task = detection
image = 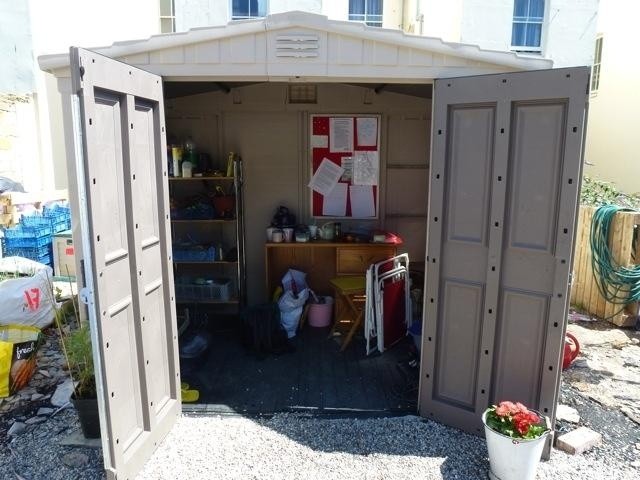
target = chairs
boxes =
[366,253,412,355]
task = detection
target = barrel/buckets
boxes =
[308,296,332,327]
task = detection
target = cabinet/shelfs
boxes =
[265,230,397,332]
[169,160,246,304]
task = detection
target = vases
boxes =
[482,406,551,480]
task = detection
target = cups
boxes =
[272,230,285,243]
[283,228,293,242]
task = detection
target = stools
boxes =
[329,277,366,353]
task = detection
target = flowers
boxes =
[487,400,548,438]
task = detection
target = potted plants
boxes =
[61,320,101,439]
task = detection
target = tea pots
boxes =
[318,222,333,239]
